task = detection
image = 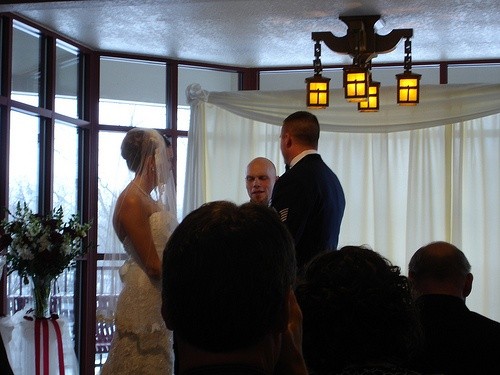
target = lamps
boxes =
[304,16,422,113]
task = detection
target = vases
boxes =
[33,287,51,318]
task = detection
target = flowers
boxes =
[184,83,210,107]
[0,202,94,286]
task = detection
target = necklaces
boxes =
[130,178,158,206]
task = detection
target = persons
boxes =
[409,242,499,375]
[245,157,281,208]
[295,246,427,374]
[269,111,346,277]
[99,126,179,375]
[160,202,309,375]
